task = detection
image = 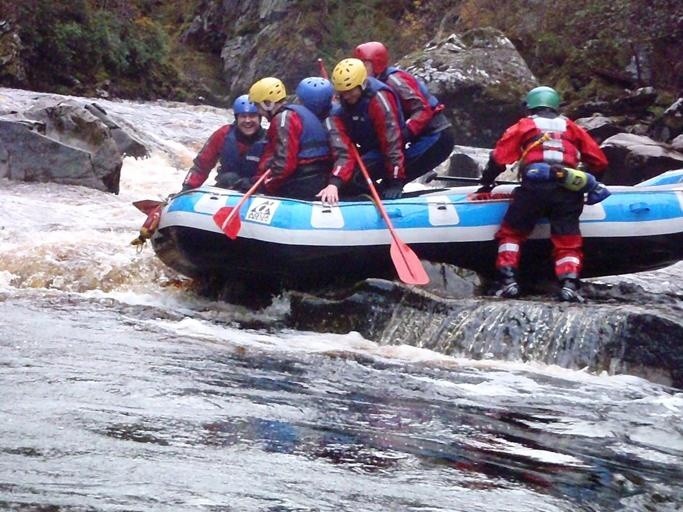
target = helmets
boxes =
[248,77,287,103]
[295,76,334,117]
[355,42,389,74]
[526,85,560,111]
[331,57,369,91]
[232,94,258,115]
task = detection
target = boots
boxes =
[560,278,585,303]
[481,266,520,298]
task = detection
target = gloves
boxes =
[381,179,403,199]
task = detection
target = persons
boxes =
[164,94,271,199]
[477,86,608,304]
[355,42,456,200]
[332,58,406,201]
[248,77,334,201]
[296,77,357,204]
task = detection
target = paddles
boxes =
[132,200,163,217]
[356,154,430,285]
[212,168,272,240]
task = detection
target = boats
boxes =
[147,166,683,297]
